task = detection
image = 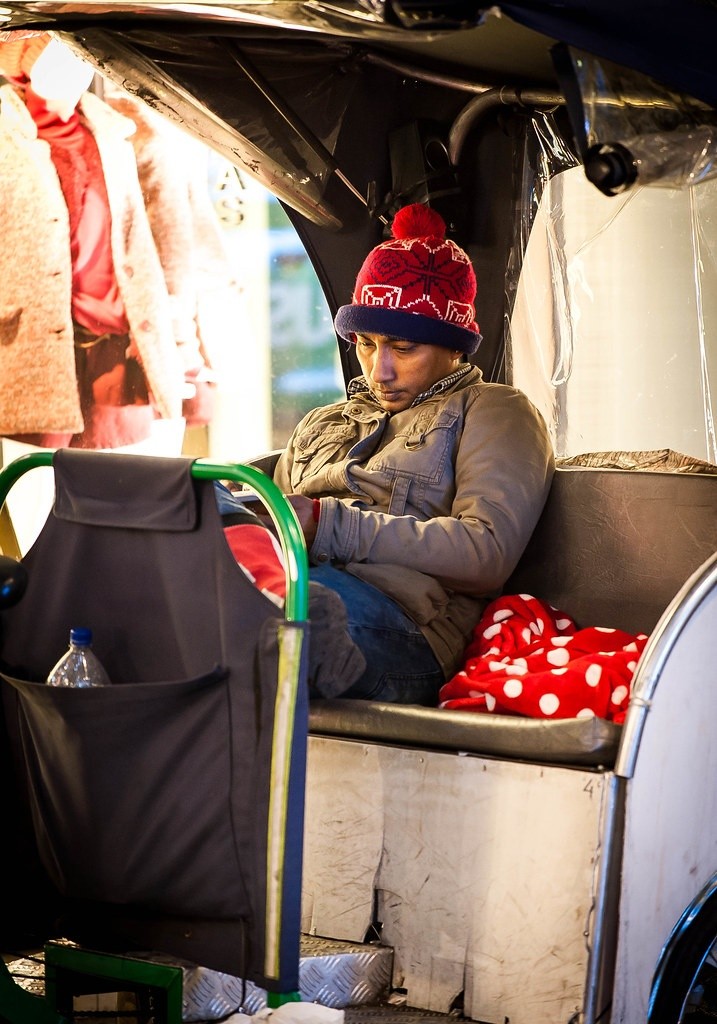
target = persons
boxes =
[223,201,555,705]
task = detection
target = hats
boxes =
[334,204,483,355]
[0,27,53,79]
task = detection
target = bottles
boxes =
[45,627,109,686]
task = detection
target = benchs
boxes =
[305,470,716,763]
